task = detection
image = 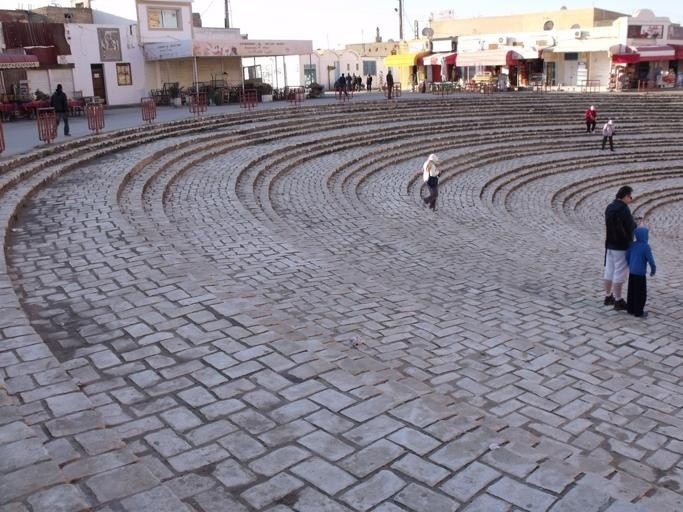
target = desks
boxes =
[0,99,82,121]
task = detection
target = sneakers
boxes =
[603,295,613,306]
[614,299,627,310]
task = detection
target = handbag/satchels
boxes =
[427,176,439,188]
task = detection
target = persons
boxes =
[386,70,392,99]
[33,90,49,118]
[50,84,72,136]
[337,73,372,97]
[584,105,597,135]
[600,119,616,151]
[625,226,657,318]
[422,154,441,210]
[603,186,641,310]
